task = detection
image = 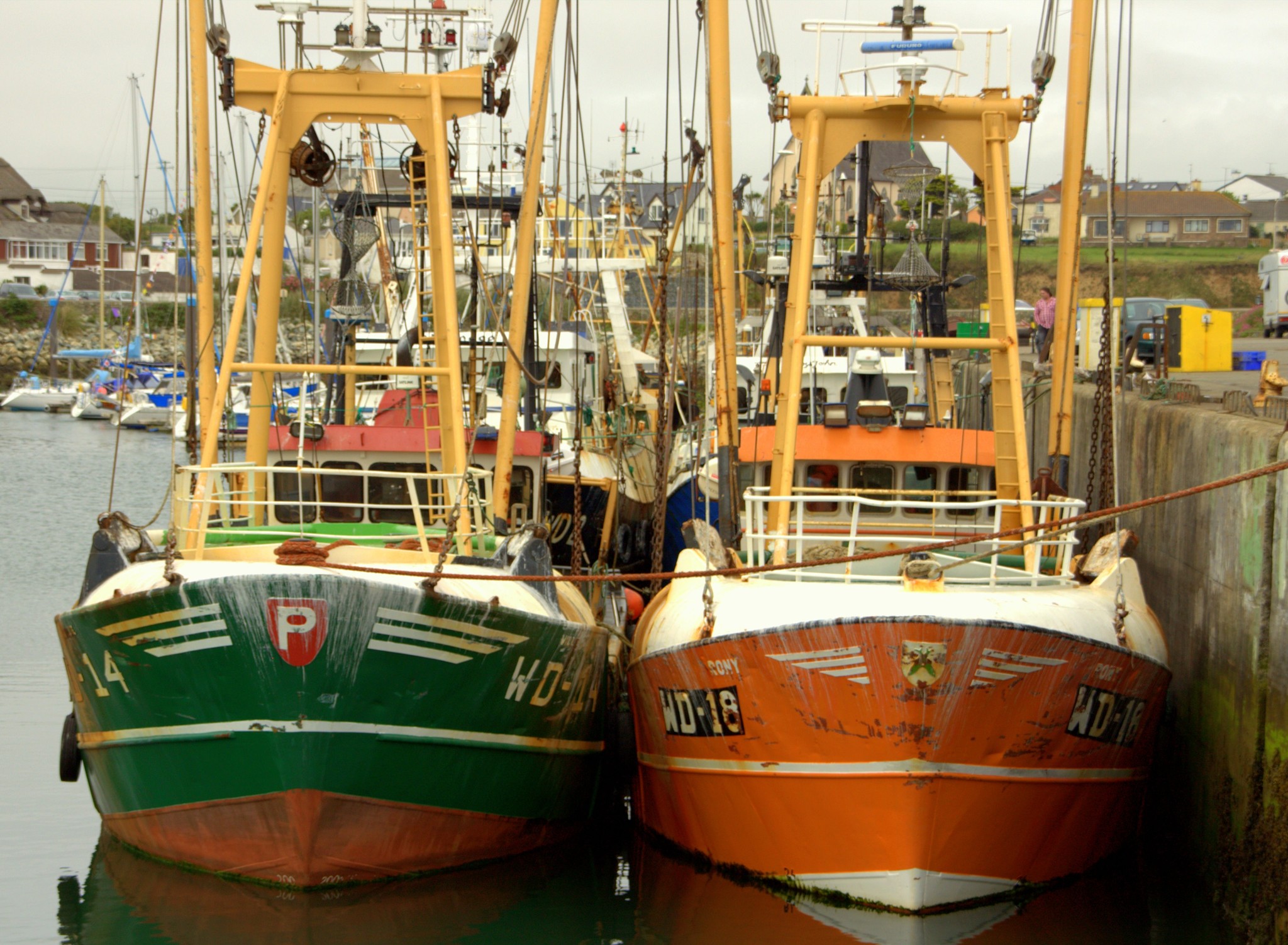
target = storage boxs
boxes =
[1233,351,1266,371]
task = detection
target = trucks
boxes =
[1257,249,1288,339]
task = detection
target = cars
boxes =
[1168,298,1211,310]
[1019,229,1037,246]
[1014,298,1039,339]
[1118,298,1175,367]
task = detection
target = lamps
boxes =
[821,402,849,426]
[742,270,764,284]
[850,153,856,170]
[474,424,497,438]
[334,25,350,45]
[901,403,930,428]
[737,364,755,386]
[949,274,978,288]
[289,421,324,441]
[366,26,382,45]
[856,400,891,424]
[826,289,843,296]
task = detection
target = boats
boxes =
[622,0,1174,915]
[49,0,650,890]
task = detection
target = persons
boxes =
[1034,287,1057,362]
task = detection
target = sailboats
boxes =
[0,71,928,584]
